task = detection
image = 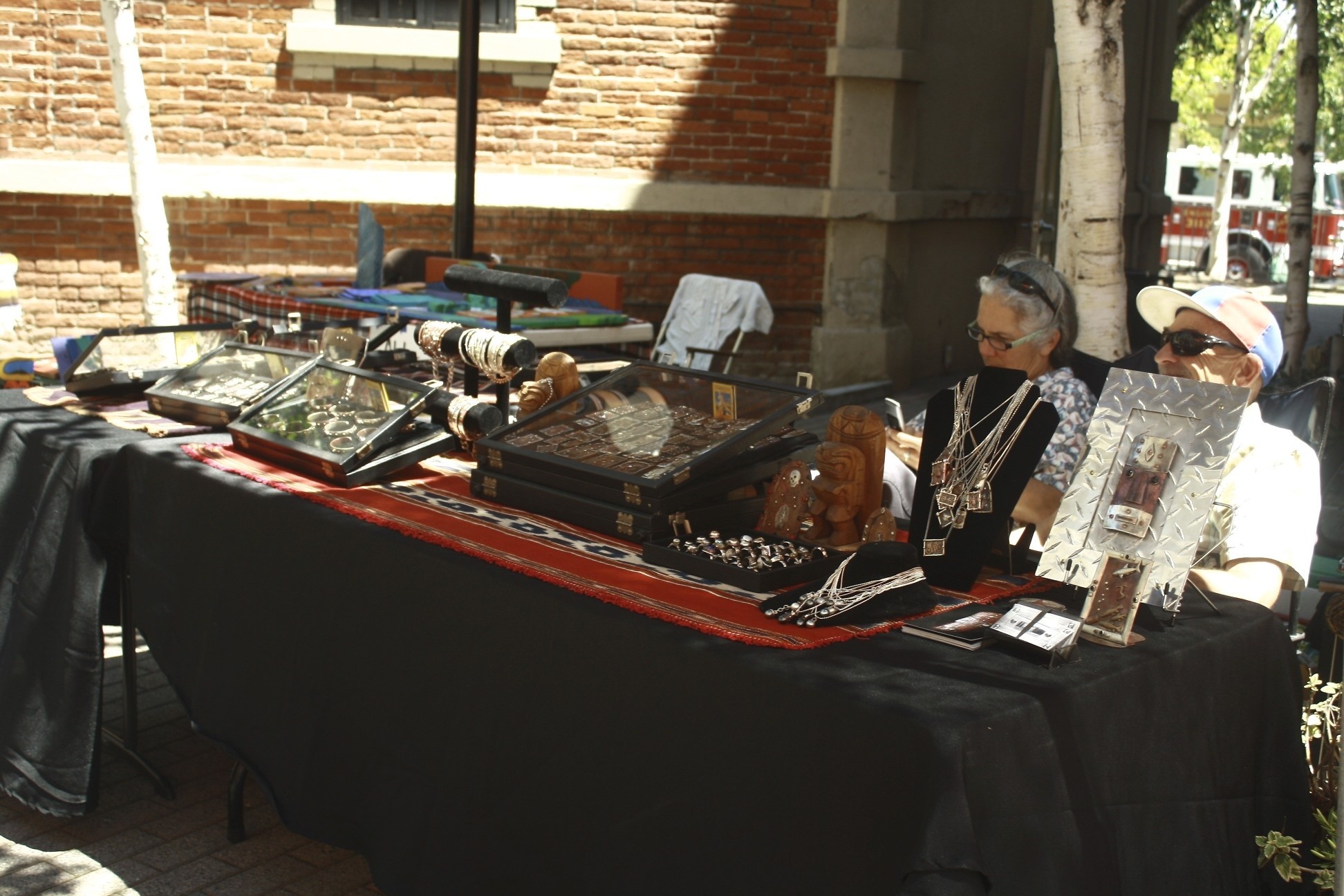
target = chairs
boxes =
[1062,343,1344,806]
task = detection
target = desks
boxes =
[96,434,1308,896]
[187,282,655,356]
[1,381,220,822]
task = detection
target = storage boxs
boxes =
[63,320,859,594]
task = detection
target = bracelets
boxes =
[418,318,528,392]
[253,396,387,453]
[447,395,487,450]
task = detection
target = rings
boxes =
[668,531,828,572]
[903,451,909,463]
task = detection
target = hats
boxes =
[1136,284,1285,388]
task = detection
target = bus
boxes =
[1156,144,1344,289]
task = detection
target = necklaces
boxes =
[765,552,926,629]
[922,375,1042,556]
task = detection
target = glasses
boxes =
[966,321,1053,352]
[983,263,1058,318]
[1159,328,1249,356]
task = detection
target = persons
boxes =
[1136,283,1322,608]
[883,249,1097,546]
